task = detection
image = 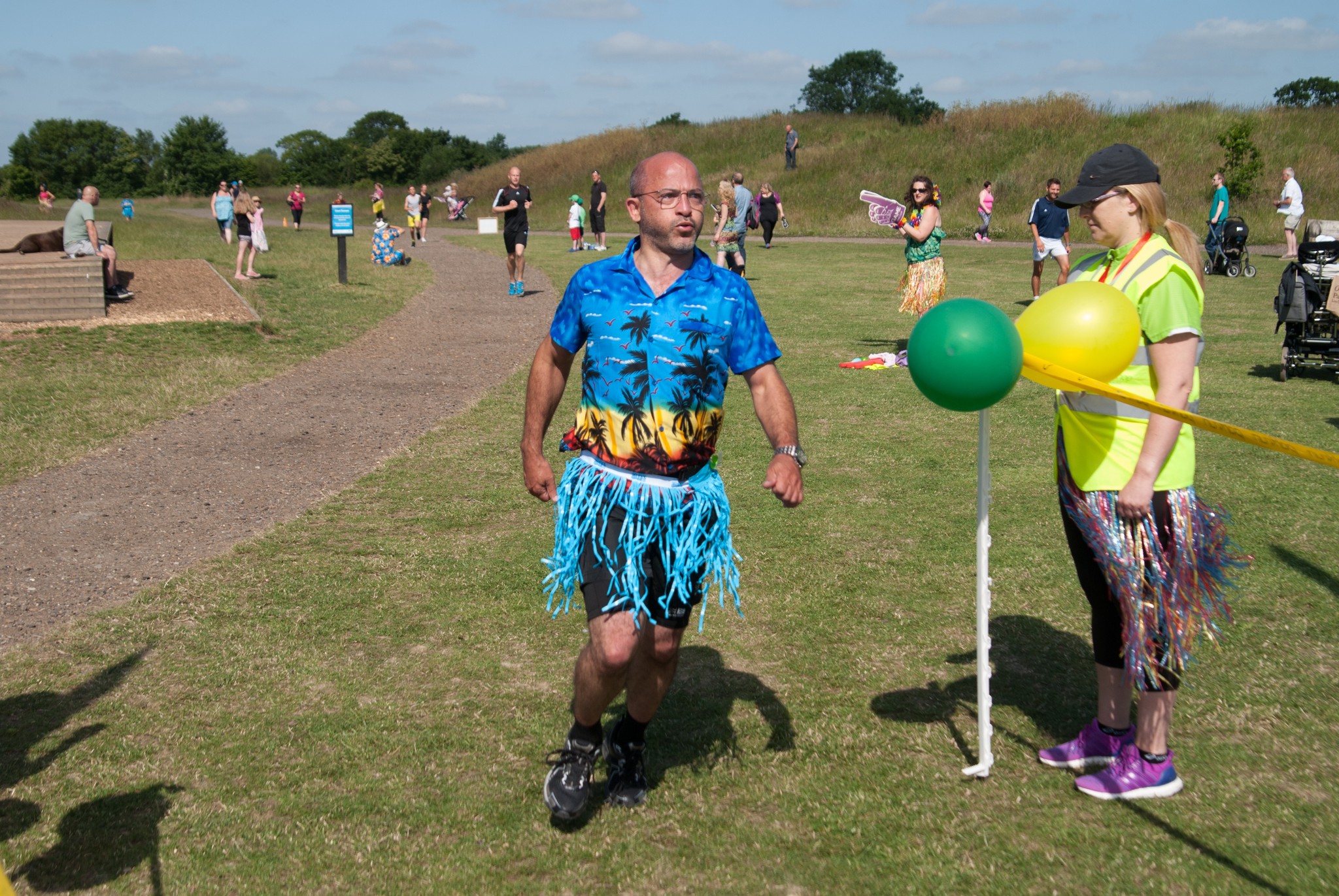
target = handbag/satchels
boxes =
[250,217,268,252]
[1273,274,1313,322]
[286,193,294,206]
[372,199,385,214]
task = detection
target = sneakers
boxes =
[600,723,647,807]
[515,281,524,296]
[975,232,982,241]
[1279,254,1294,260]
[105,281,134,298]
[509,282,516,295]
[982,237,991,242]
[1073,743,1183,799]
[544,723,605,818]
[1293,254,1298,259]
[1038,717,1138,767]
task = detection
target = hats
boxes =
[230,180,262,203]
[374,220,388,232]
[1054,142,1162,209]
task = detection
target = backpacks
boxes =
[746,191,761,229]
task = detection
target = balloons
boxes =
[907,297,1023,412]
[1015,280,1141,393]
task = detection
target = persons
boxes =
[975,181,995,241]
[1273,167,1305,260]
[38,183,136,298]
[1205,172,1229,272]
[784,124,799,170]
[1027,178,1072,300]
[589,170,607,251]
[491,166,532,297]
[211,179,464,280]
[521,152,806,820]
[1036,143,1247,801]
[711,172,784,279]
[859,175,946,321]
[567,194,586,252]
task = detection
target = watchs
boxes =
[1066,242,1071,245]
[774,445,807,468]
[95,248,100,253]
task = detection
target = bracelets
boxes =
[1278,199,1280,204]
[899,217,908,228]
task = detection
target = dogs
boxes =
[0,226,65,256]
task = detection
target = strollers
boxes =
[1272,240,1339,381]
[1203,215,1257,278]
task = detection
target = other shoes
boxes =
[373,220,378,224]
[246,270,261,277]
[234,273,248,279]
[766,243,771,248]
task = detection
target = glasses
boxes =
[1213,178,1221,181]
[220,184,227,186]
[634,188,710,206]
[1082,189,1129,208]
[911,188,930,193]
